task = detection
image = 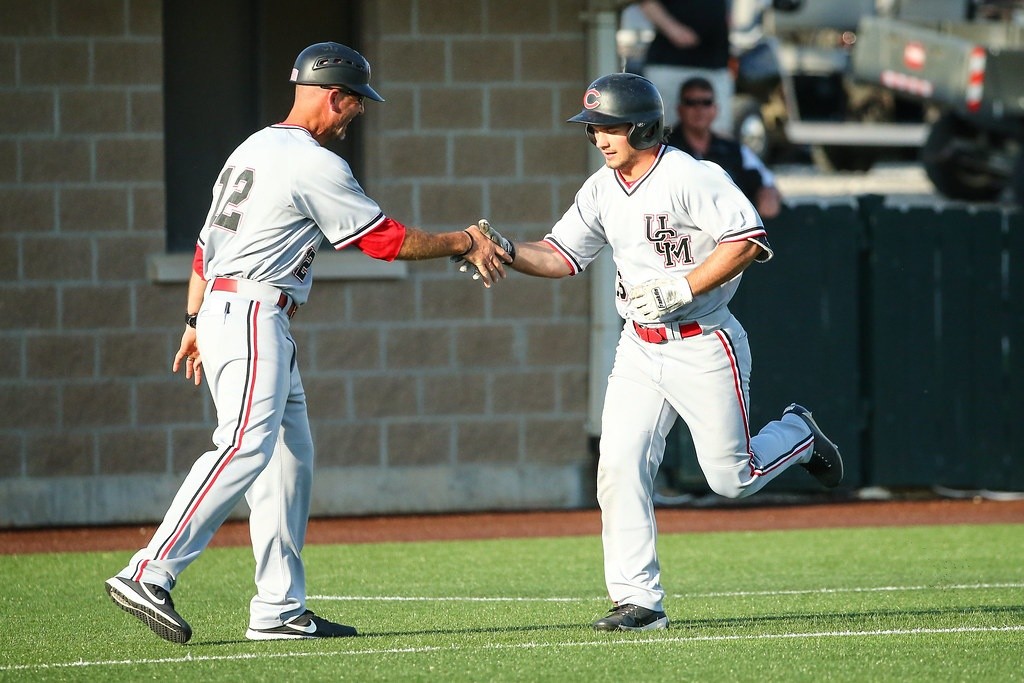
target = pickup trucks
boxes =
[848,11,1024,201]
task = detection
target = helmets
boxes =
[567,72,664,149]
[289,41,384,106]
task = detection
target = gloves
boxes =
[448,218,514,281]
[629,277,696,320]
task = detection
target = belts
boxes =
[211,278,296,318]
[633,321,700,344]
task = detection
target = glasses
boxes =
[683,98,713,108]
[318,81,366,104]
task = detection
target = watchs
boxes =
[185,311,198,329]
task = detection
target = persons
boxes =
[105,41,513,644]
[640,1,735,137]
[664,76,781,215]
[449,74,843,630]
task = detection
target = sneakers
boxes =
[783,404,843,487]
[244,613,356,642]
[595,601,669,632]
[105,576,192,644]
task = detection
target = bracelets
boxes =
[457,230,474,256]
